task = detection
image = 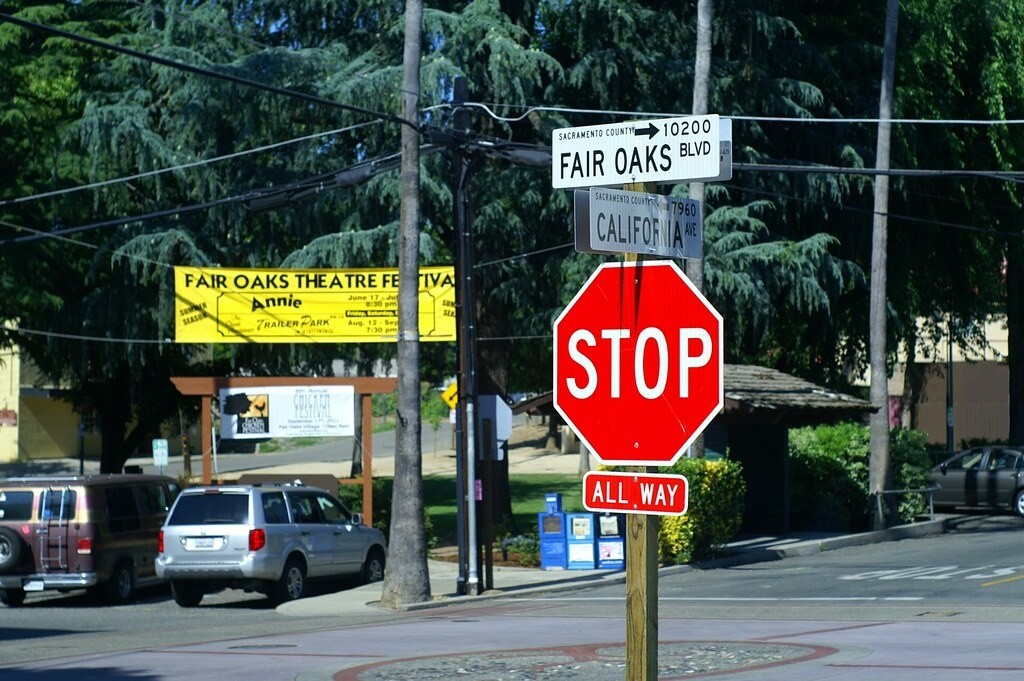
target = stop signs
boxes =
[552,259,725,467]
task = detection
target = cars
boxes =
[923,444,1024,519]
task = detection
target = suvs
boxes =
[157,480,388,609]
[0,473,184,607]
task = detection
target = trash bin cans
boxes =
[538,512,624,569]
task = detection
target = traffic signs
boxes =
[572,186,705,259]
[551,114,720,189]
